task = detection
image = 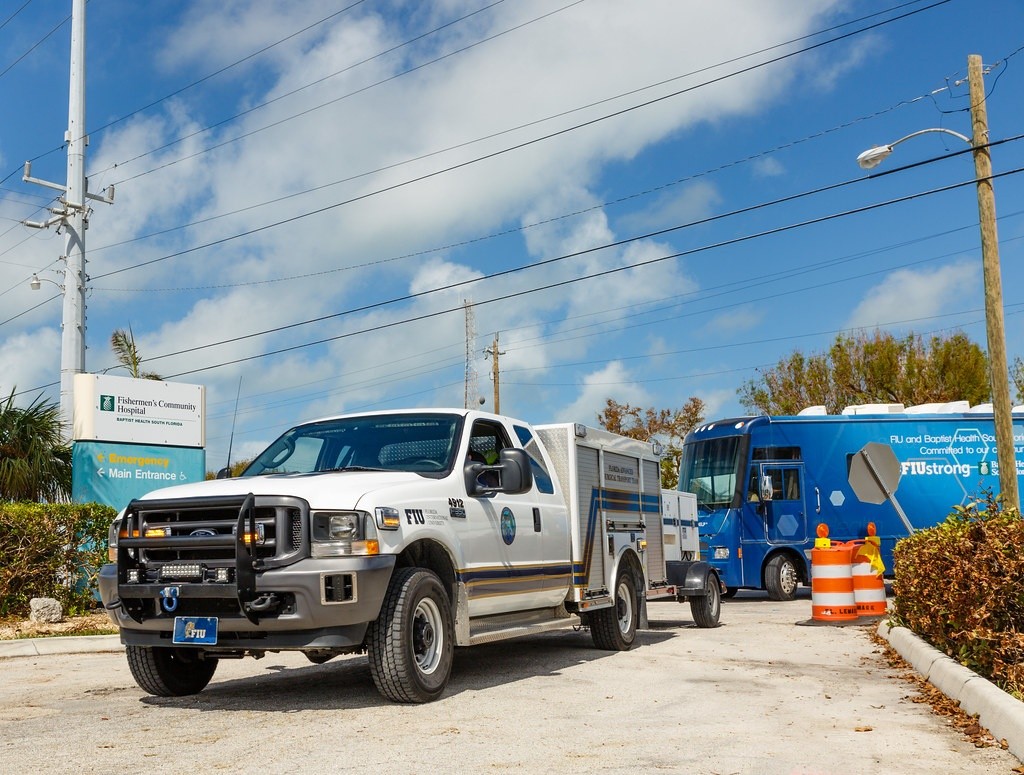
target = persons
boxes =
[437,443,488,487]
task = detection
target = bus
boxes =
[677,392,1024,601]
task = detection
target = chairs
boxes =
[471,451,499,498]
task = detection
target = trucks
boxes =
[94,409,725,704]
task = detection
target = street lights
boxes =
[856,128,1023,515]
